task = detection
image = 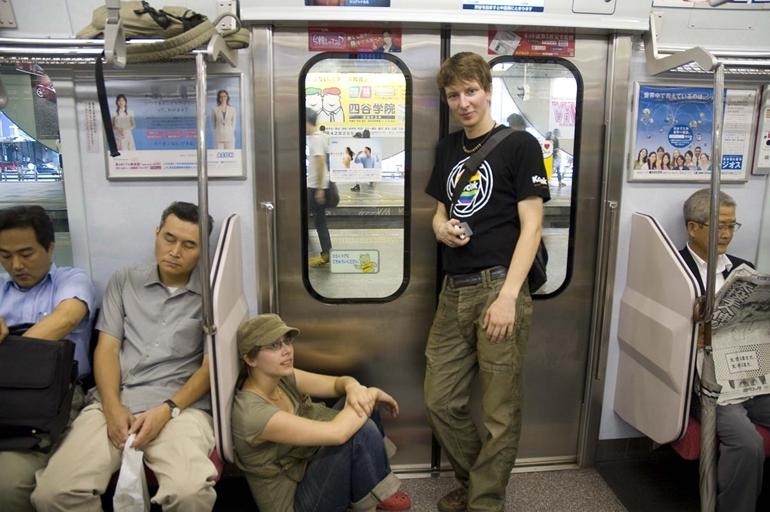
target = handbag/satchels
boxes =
[322,180,339,209]
[527,238,549,291]
[0,323,79,453]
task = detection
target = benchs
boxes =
[673,413,769,463]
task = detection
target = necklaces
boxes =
[460,124,495,154]
[249,376,284,403]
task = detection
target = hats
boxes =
[237,313,300,356]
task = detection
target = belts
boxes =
[446,265,506,288]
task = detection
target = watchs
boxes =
[164,399,180,418]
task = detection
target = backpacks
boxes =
[76,0,250,63]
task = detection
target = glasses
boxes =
[705,223,741,232]
[261,335,295,350]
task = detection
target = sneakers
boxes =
[308,257,329,266]
[377,486,468,512]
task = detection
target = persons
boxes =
[421,50,551,510]
[212,90,235,150]
[633,146,713,171]
[340,142,356,170]
[323,87,345,123]
[28,199,219,512]
[350,129,372,191]
[354,147,381,169]
[377,30,398,51]
[676,188,770,512]
[0,203,97,512]
[545,126,567,188]
[305,88,327,124]
[111,94,137,150]
[304,107,333,269]
[231,313,412,512]
[507,110,528,132]
[318,122,331,173]
